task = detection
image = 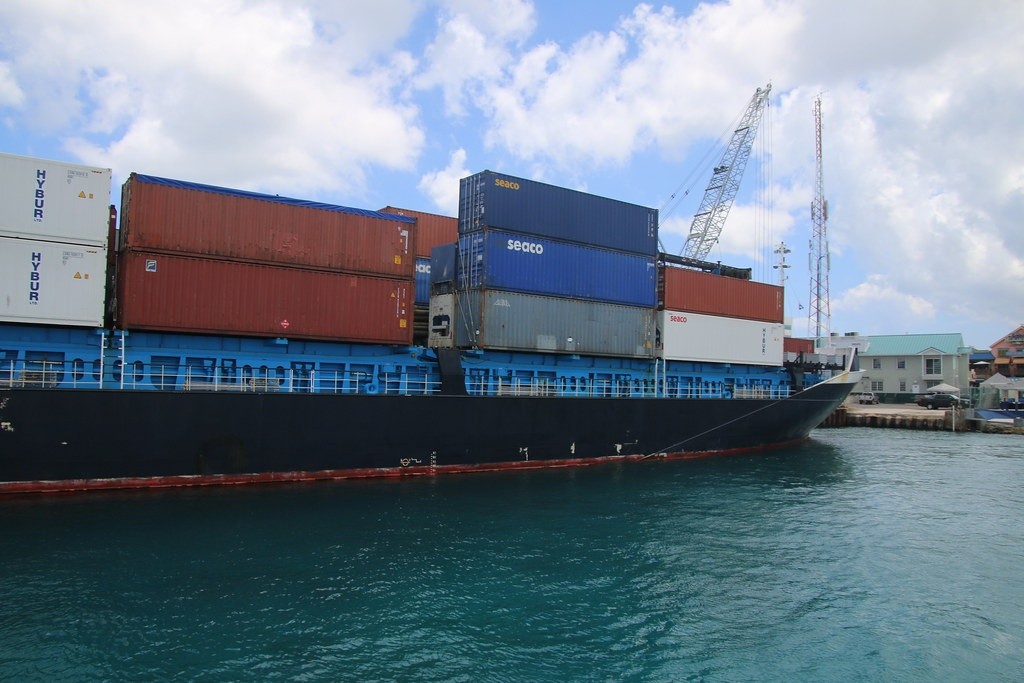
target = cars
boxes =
[859,390,879,404]
[914,393,970,410]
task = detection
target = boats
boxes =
[2,82,869,498]
